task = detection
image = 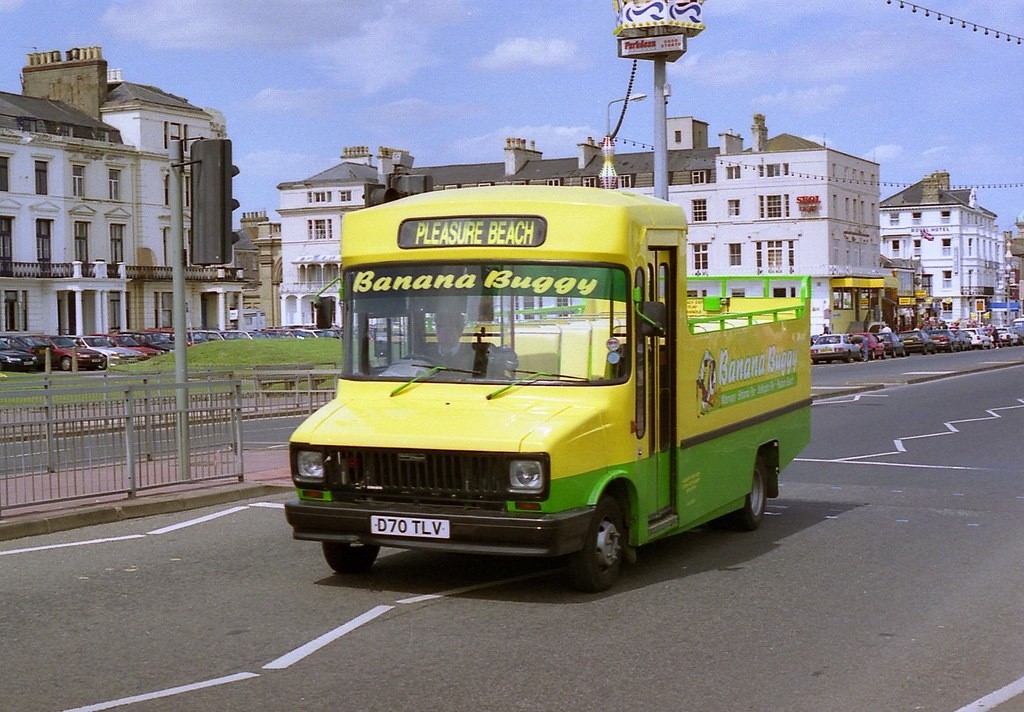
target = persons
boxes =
[882,324,891,333]
[990,325,1001,349]
[822,323,832,333]
[1003,319,1010,327]
[862,333,868,362]
[417,311,475,381]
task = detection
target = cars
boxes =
[897,330,936,356]
[0,319,497,373]
[927,330,961,353]
[952,330,972,351]
[874,333,906,358]
[849,333,887,360]
[983,326,1003,349]
[811,334,863,363]
[995,327,1020,347]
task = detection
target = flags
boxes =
[920,229,934,241]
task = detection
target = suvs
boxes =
[961,328,991,350]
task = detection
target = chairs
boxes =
[424,341,498,379]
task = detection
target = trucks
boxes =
[284,185,814,592]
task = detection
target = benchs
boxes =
[471,310,797,380]
